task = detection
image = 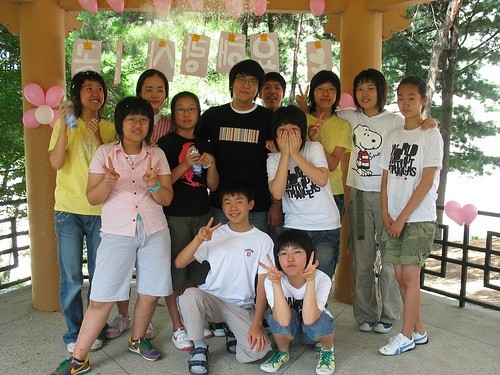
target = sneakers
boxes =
[105,314,132,339]
[88,339,104,352]
[260,351,290,373]
[388,330,427,344]
[203,328,213,338]
[128,336,162,361]
[223,322,237,353]
[376,333,415,355]
[188,345,209,375]
[374,319,393,333]
[358,321,374,332]
[47,355,91,375]
[171,326,194,351]
[66,343,75,354]
[316,344,336,375]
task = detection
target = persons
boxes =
[257,230,336,375]
[50,97,174,375]
[105,69,172,340]
[295,69,439,334]
[47,70,120,355]
[194,59,285,336]
[157,91,222,352]
[265,69,354,222]
[378,73,444,356]
[266,105,342,351]
[175,181,276,375]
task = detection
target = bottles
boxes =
[61,100,78,130]
[189,143,201,174]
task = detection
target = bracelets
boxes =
[147,183,161,192]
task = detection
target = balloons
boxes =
[460,205,478,226]
[49,110,61,129]
[45,86,65,108]
[78,0,97,13]
[337,92,356,110]
[309,0,325,16]
[35,105,54,125]
[154,0,171,18]
[225,0,243,18]
[445,201,463,227]
[250,0,268,16]
[189,0,203,11]
[23,83,45,107]
[23,108,40,128]
[107,0,124,12]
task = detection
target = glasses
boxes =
[314,87,336,93]
[235,76,258,86]
[123,117,150,124]
[171,107,199,112]
